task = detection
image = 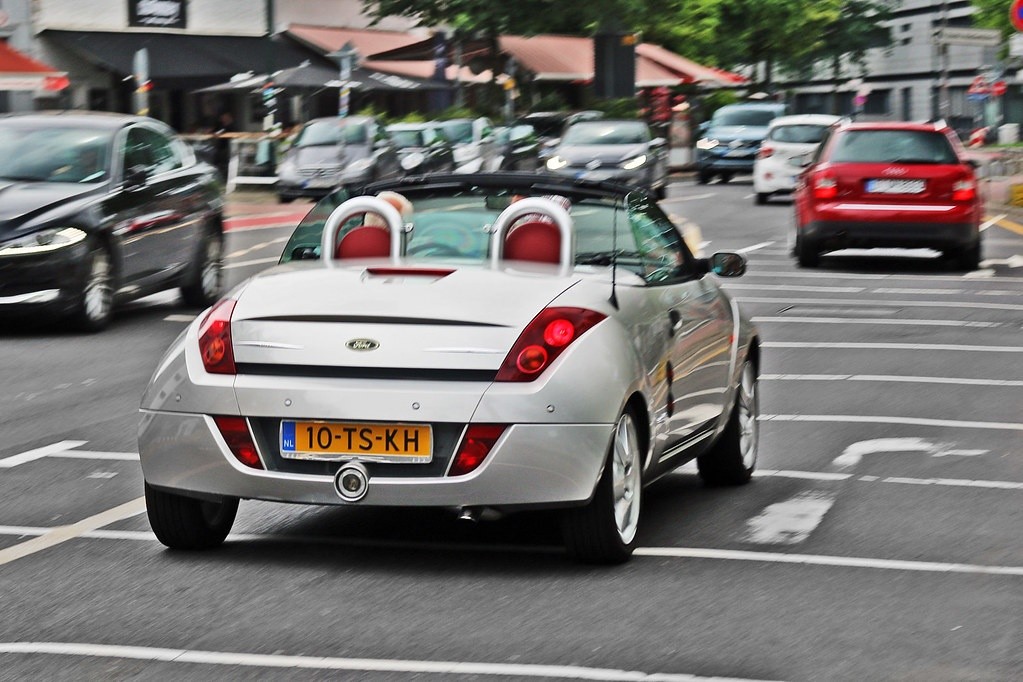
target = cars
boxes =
[1,109,224,327]
[794,120,982,271]
[543,121,673,202]
[691,102,794,183]
[443,103,606,193]
[384,123,456,196]
[754,113,846,215]
[275,119,399,199]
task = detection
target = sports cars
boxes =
[139,170,764,567]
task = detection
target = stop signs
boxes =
[993,81,1006,96]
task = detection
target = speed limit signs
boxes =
[971,75,989,95]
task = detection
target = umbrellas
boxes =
[196,58,450,125]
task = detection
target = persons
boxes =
[215,109,235,165]
[365,189,416,248]
